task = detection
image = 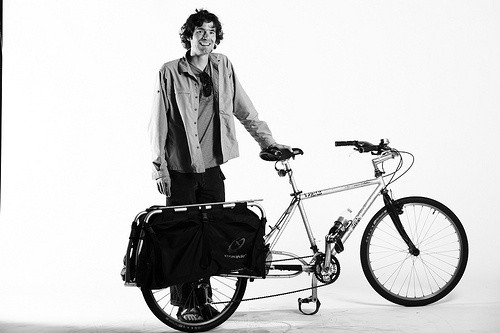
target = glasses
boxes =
[200,72,213,98]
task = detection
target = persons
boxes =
[151,8,296,324]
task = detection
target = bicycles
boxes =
[122,139,468,333]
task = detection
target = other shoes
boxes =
[179,305,221,322]
